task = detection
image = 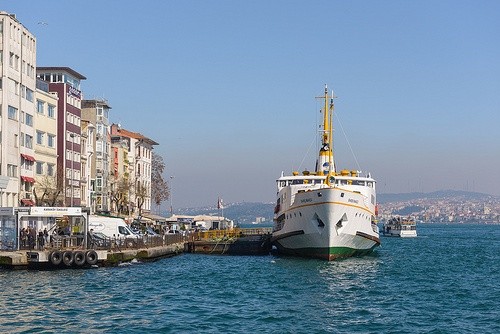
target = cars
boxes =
[164,230,186,235]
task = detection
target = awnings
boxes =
[21,175,35,183]
[20,197,35,206]
[21,153,36,161]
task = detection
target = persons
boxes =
[90,229,93,231]
[20,225,80,248]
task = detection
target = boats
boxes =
[190,197,242,252]
[385,215,417,238]
[266,84,381,259]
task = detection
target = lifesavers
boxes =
[86,250,98,264]
[74,251,85,266]
[49,251,63,265]
[63,251,74,266]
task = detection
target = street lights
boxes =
[70,132,75,237]
[169,175,174,215]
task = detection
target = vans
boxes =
[88,215,161,242]
[87,232,115,247]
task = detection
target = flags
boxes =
[218,196,223,209]
